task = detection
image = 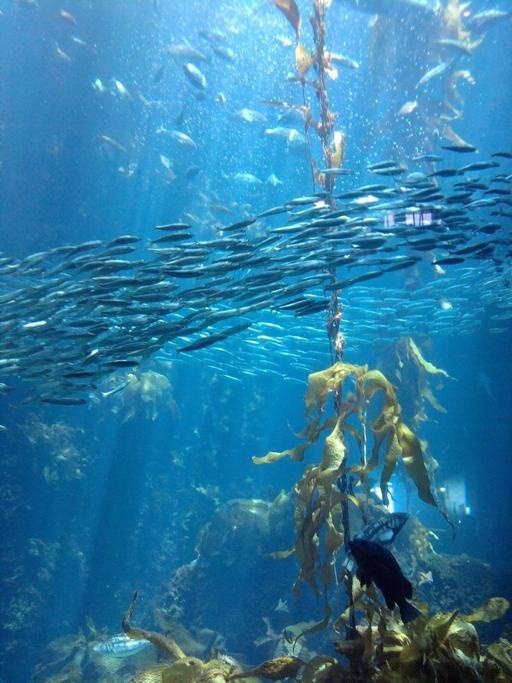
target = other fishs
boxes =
[1,0,512,406]
